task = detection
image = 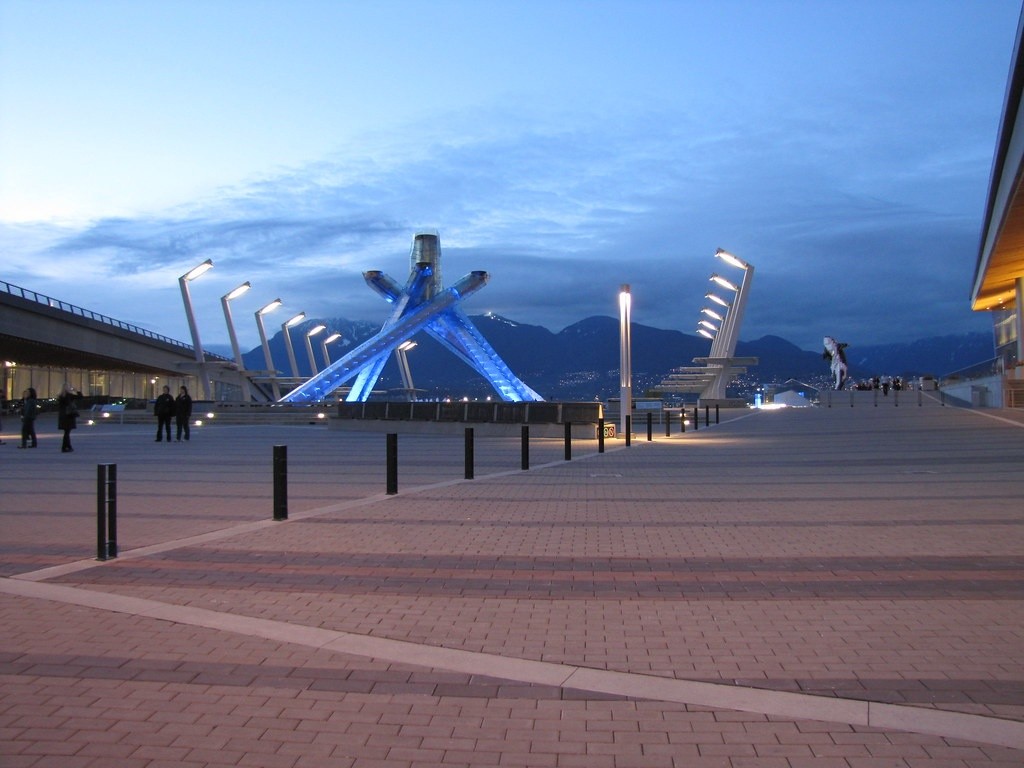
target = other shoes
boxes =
[182,438,188,441]
[0,442,5,445]
[70,448,73,451]
[63,449,71,452]
[155,439,161,442]
[174,439,181,442]
[28,445,37,448]
[17,446,27,449]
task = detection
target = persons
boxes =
[873,376,880,389]
[173,386,192,442]
[881,373,891,396]
[12,388,37,449]
[893,376,901,390]
[56,384,83,452]
[153,386,174,442]
[0,438,6,445]
[822,336,849,389]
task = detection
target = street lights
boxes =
[180,260,211,400]
[393,338,420,402]
[322,332,343,370]
[255,298,283,399]
[697,247,754,408]
[616,282,638,438]
[282,312,306,379]
[305,325,321,377]
[219,281,253,403]
